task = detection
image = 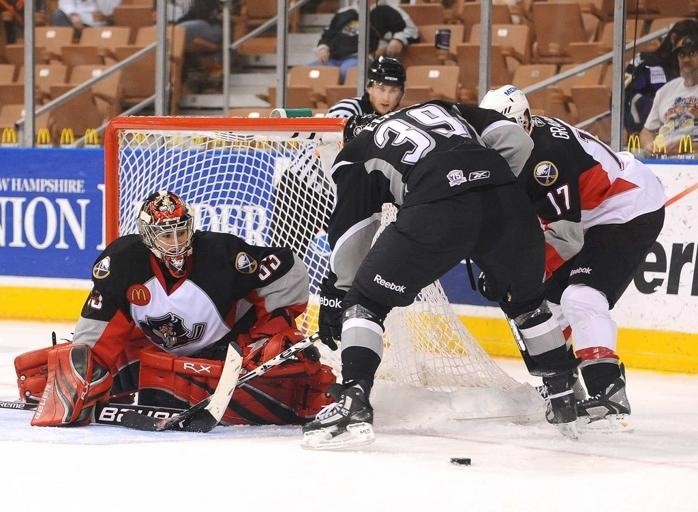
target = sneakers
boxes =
[301,379,374,435]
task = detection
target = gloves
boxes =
[316,273,356,352]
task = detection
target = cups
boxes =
[435,28,450,49]
[270,107,313,117]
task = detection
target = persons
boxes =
[68,189,310,360]
[303,1,424,86]
[324,54,407,118]
[622,19,698,154]
[300,97,591,439]
[47,0,126,43]
[2,1,40,44]
[479,83,667,423]
[147,1,245,52]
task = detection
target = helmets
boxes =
[476,82,533,123]
[342,111,378,147]
[136,188,196,236]
[363,54,407,89]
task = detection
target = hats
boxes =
[668,33,698,55]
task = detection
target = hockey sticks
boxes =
[1,341,244,432]
[121,329,321,432]
[506,315,583,377]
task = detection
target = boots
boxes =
[538,356,586,426]
[576,357,630,418]
